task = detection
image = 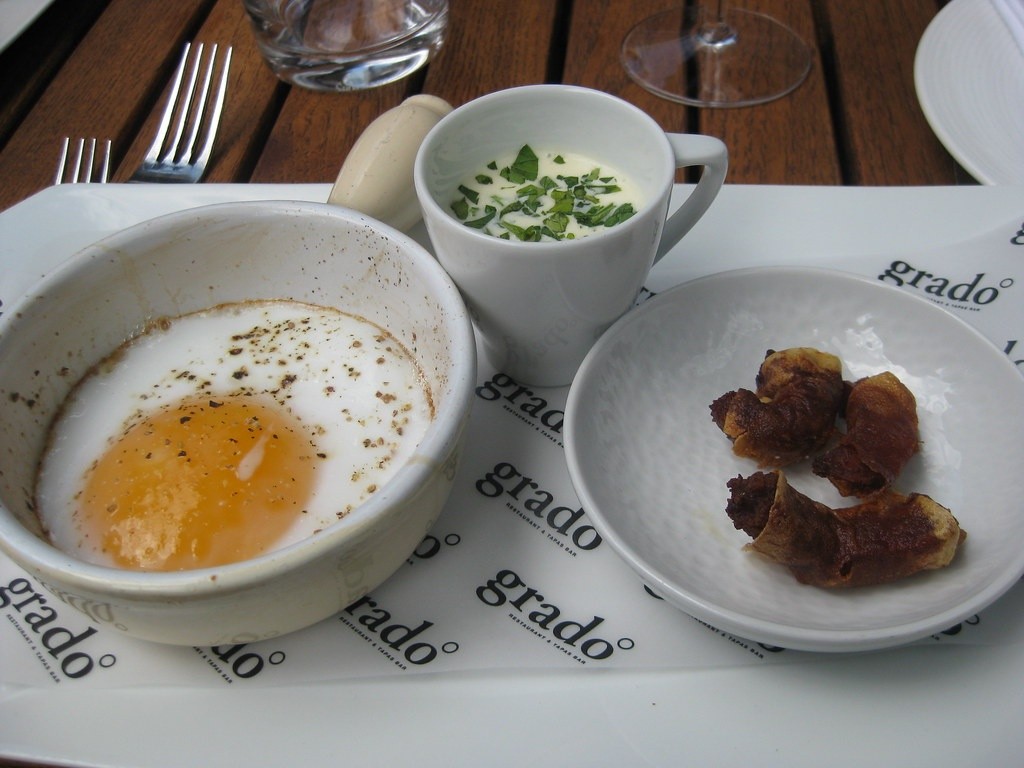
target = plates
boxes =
[563,263,1024,650]
[914,0,1024,185]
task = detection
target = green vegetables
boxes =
[449,142,639,243]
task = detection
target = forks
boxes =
[127,42,233,183]
[55,137,111,184]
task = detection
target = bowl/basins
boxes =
[0,92,477,645]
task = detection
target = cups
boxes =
[242,0,449,93]
[414,85,727,387]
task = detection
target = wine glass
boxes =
[619,0,812,109]
